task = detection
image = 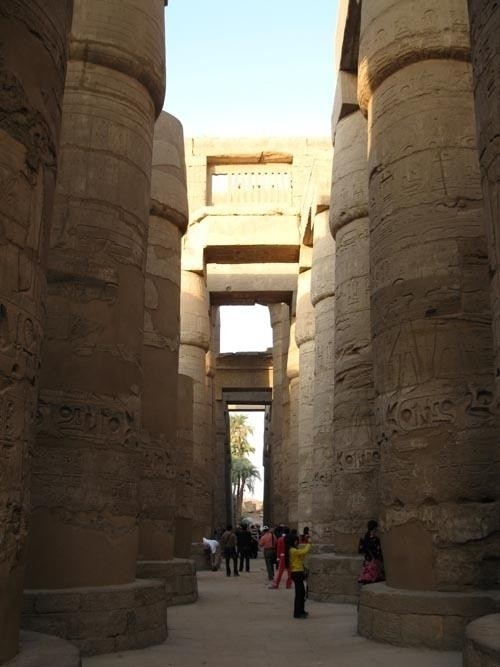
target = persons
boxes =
[203,521,309,590]
[357,520,386,584]
[283,533,312,618]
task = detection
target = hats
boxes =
[261,526,270,533]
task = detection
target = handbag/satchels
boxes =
[270,550,276,564]
